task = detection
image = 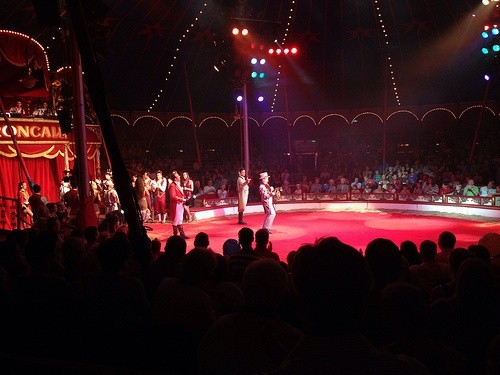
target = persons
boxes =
[56,96,64,110]
[38,102,51,116]
[9,101,25,114]
[0,146,500,375]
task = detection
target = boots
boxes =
[173,226,189,239]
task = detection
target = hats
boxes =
[258,172,270,179]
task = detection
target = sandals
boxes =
[238,212,247,224]
